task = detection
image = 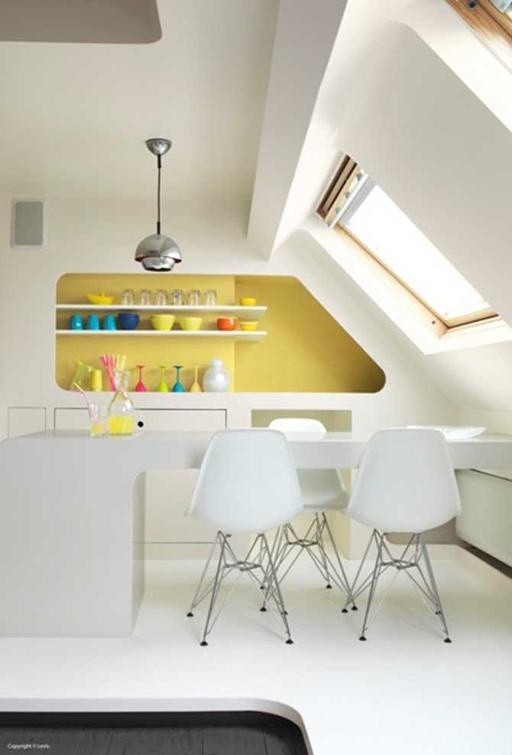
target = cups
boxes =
[86,314,102,329]
[204,290,217,306]
[169,288,183,305]
[121,289,138,305]
[70,314,85,329]
[187,289,201,305]
[140,289,151,306]
[90,370,103,393]
[103,314,117,329]
[155,289,167,306]
[87,399,107,437]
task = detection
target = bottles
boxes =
[106,371,136,437]
[202,360,230,392]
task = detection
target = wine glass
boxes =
[135,364,148,391]
[190,364,203,392]
[156,364,170,391]
[171,365,186,392]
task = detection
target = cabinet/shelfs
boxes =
[54,305,267,340]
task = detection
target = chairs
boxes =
[189,432,304,646]
[345,428,460,643]
[256,417,360,615]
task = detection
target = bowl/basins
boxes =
[217,317,237,330]
[239,297,256,306]
[149,315,176,329]
[238,322,257,330]
[177,317,202,329]
[117,313,139,330]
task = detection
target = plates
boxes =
[85,294,116,305]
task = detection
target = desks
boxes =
[0,432,512,637]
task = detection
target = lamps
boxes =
[132,139,183,273]
